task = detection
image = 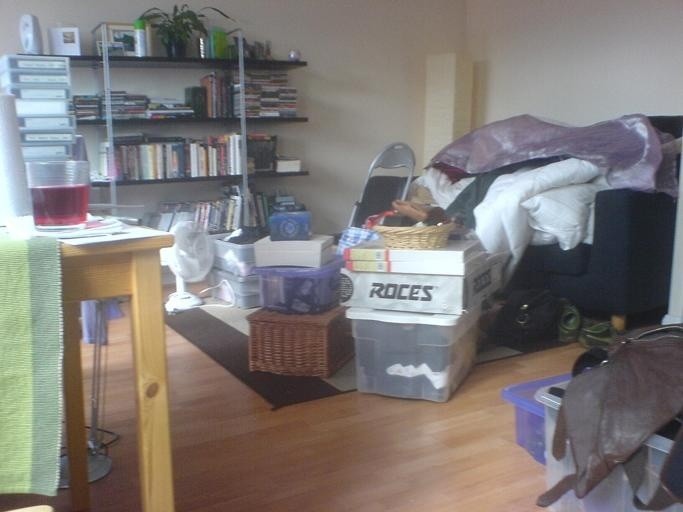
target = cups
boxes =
[23,160,90,228]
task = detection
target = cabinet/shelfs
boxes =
[18,23,310,235]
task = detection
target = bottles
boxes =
[132,19,148,56]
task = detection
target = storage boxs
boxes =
[534,379,683,506]
[339,262,504,315]
[344,303,484,405]
[251,255,346,315]
[499,370,595,465]
[212,234,272,275]
[212,267,262,311]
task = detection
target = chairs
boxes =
[345,142,416,231]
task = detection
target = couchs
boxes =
[508,188,678,334]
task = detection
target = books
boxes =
[73,71,299,235]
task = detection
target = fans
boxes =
[160,221,215,311]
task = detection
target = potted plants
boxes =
[139,3,242,59]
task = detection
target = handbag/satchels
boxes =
[503,287,563,343]
[564,323,683,498]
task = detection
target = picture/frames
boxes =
[108,24,137,59]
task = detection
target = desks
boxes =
[0,216,177,512]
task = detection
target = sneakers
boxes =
[557,295,581,342]
[579,321,620,347]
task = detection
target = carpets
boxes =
[162,294,525,413]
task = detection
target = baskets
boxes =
[372,223,452,249]
